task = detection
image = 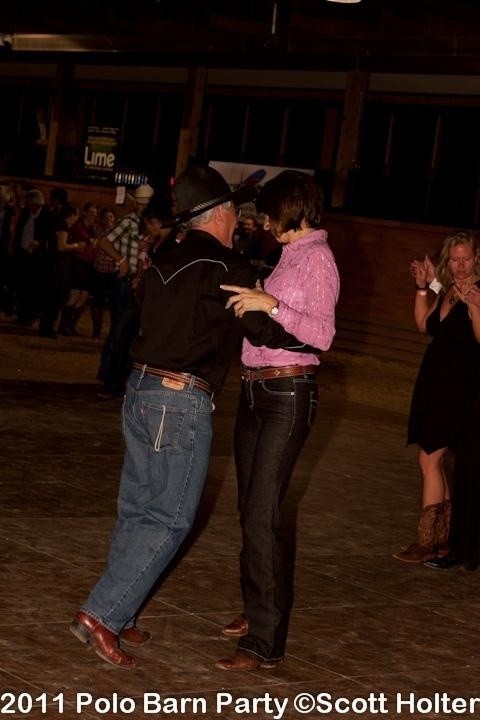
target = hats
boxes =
[26,189,44,206]
[159,164,266,229]
[126,183,154,205]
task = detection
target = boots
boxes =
[392,499,452,562]
[57,305,84,336]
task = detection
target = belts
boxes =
[132,362,217,401]
[239,364,316,381]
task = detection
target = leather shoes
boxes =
[423,555,477,571]
[69,612,135,669]
[221,613,249,637]
[118,626,152,647]
[215,650,277,671]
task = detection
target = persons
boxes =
[1,172,290,402]
[215,168,341,671]
[391,231,479,571]
[69,163,321,671]
